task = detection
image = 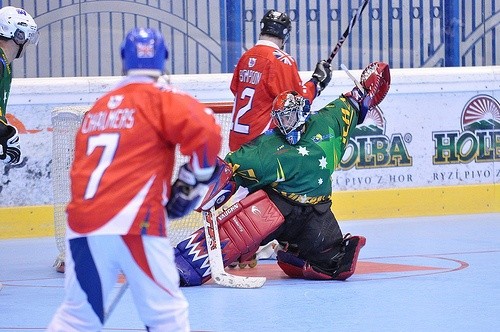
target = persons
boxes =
[0,6,39,207]
[174,9,391,261]
[173,61,392,290]
[47,26,221,332]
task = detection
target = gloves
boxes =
[0,122,23,166]
[312,59,333,87]
[166,164,210,219]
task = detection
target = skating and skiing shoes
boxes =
[227,251,258,268]
[272,243,284,255]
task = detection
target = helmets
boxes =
[271,89,311,144]
[0,6,39,46]
[260,9,292,44]
[121,27,168,76]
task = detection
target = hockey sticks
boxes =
[104,185,190,323]
[201,208,268,289]
[328,0,370,59]
